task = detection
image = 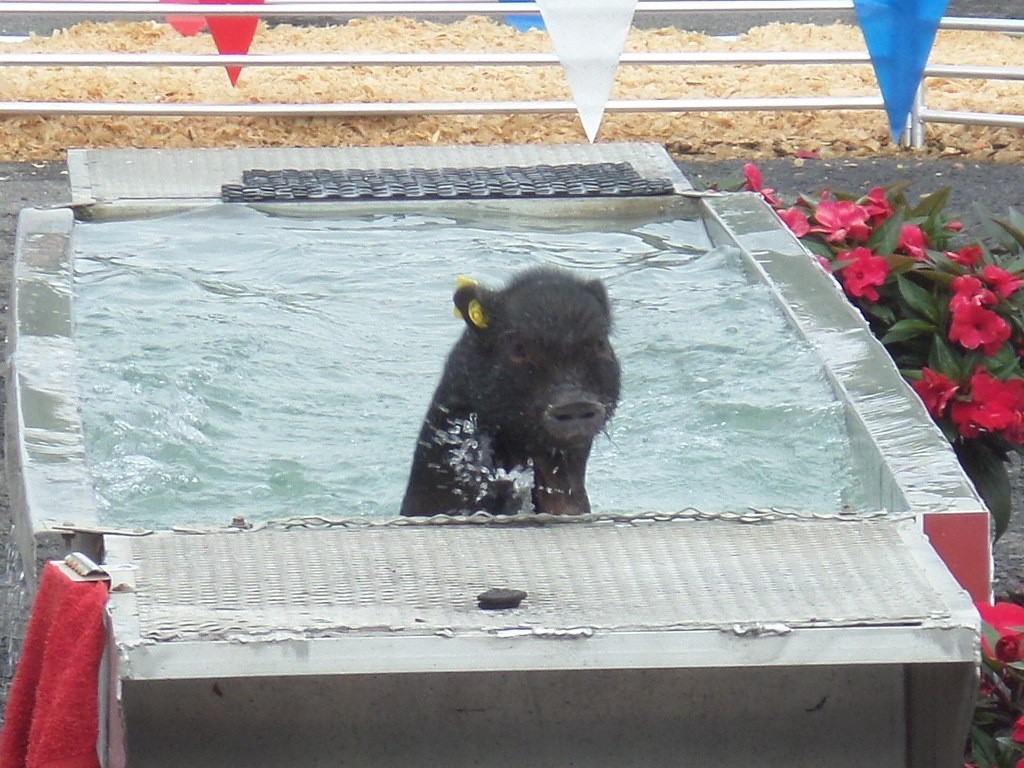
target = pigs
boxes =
[398,265,625,520]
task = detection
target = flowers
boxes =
[966,597,1024,767]
[740,154,1024,473]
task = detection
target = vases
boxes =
[970,460,1014,546]
